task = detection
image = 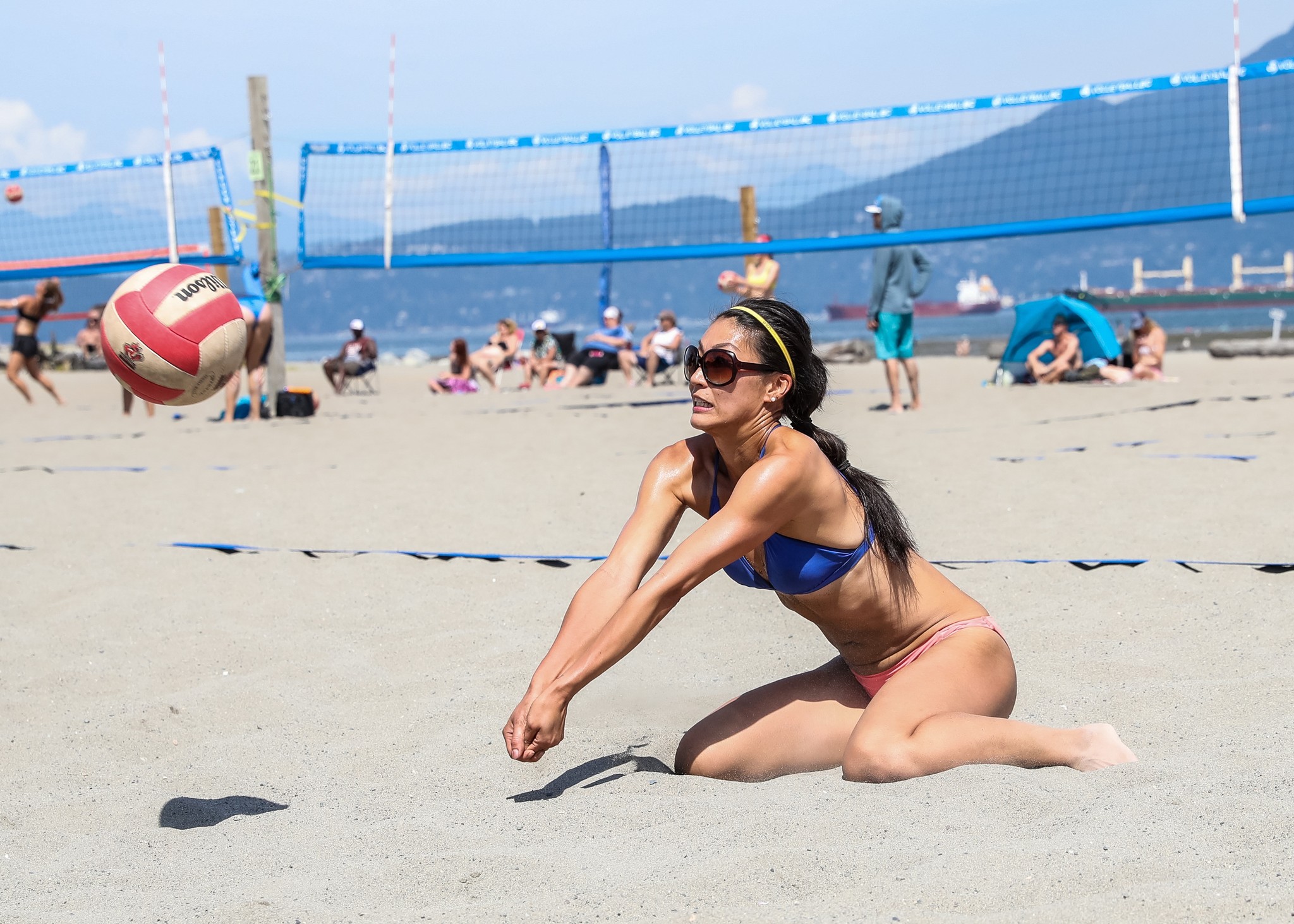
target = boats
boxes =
[955,267,1001,317]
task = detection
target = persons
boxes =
[1001,312,1168,387]
[469,318,519,388]
[124,388,155,417]
[864,194,933,413]
[427,338,473,395]
[0,276,64,407]
[517,319,566,390]
[617,310,684,390]
[75,319,105,362]
[721,233,780,300]
[559,305,635,387]
[216,296,272,422]
[324,319,377,394]
[501,290,1139,784]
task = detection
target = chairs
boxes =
[333,331,684,398]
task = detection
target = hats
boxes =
[604,305,618,318]
[349,319,364,330]
[531,319,546,330]
[865,195,881,214]
[1130,312,1143,328]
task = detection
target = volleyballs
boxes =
[99,262,248,407]
[4,183,23,204]
[718,269,740,294]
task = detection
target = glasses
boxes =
[682,344,770,387]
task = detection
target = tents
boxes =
[1001,295,1123,377]
